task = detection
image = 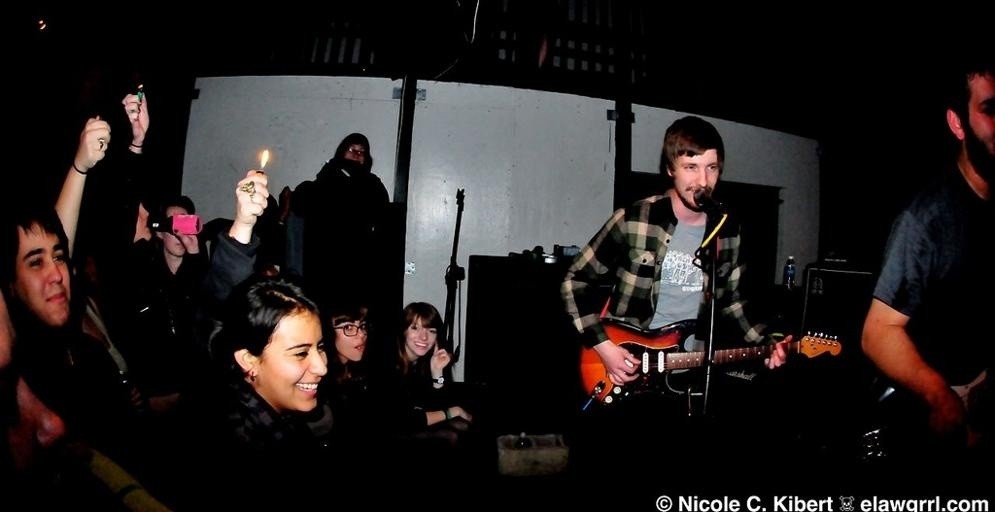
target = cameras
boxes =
[147,215,203,236]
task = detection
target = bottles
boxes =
[781,255,798,291]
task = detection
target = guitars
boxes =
[582,320,841,406]
[864,370,988,484]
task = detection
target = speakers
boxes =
[800,263,874,340]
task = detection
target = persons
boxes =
[1,81,472,511]
[857,62,993,455]
[560,114,794,407]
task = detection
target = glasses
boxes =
[348,147,365,158]
[334,323,368,336]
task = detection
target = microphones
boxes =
[693,188,740,237]
